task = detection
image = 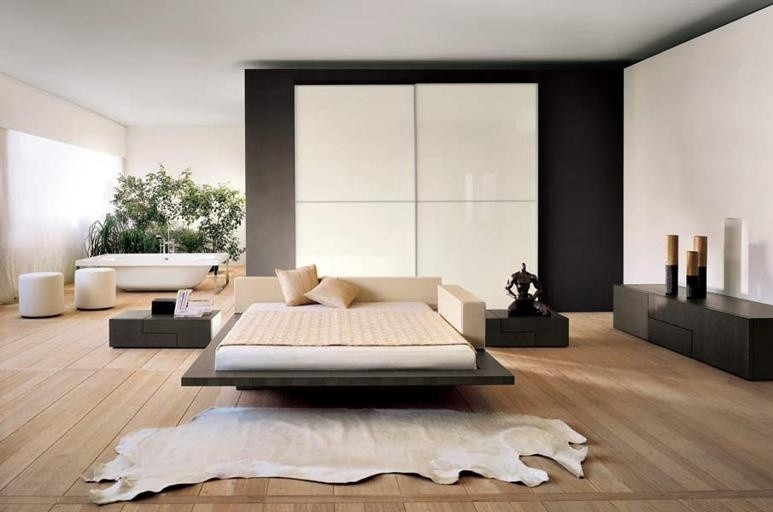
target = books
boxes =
[175,288,214,317]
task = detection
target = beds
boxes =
[181,277,515,390]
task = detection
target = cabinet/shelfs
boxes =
[613,283,773,383]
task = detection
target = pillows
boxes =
[275,265,321,306]
[303,276,360,308]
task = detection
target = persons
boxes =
[506,263,543,311]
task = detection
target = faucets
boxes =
[156,234,165,253]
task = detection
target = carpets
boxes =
[78,407,587,504]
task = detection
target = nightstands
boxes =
[485,308,570,348]
[109,310,222,349]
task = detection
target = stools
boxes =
[18,272,65,316]
[74,268,116,310]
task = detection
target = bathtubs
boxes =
[73,251,230,292]
